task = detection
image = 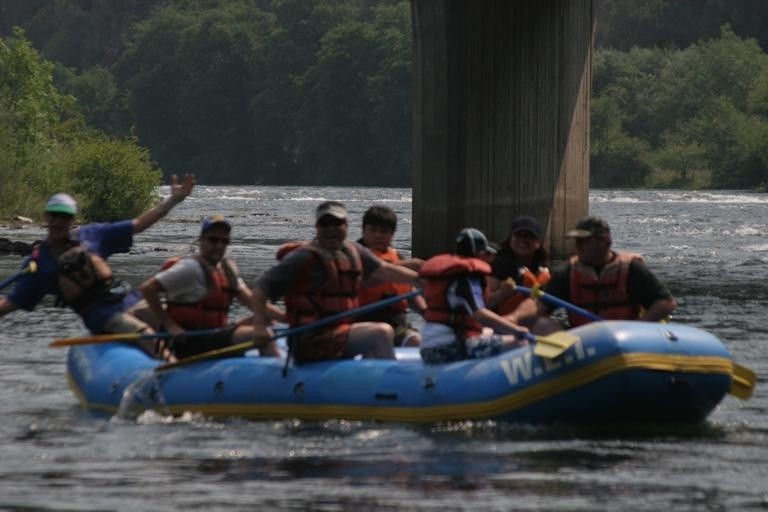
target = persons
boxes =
[418,228,529,364]
[482,216,564,335]
[501,214,676,327]
[251,202,418,359]
[0,171,196,363]
[356,206,425,347]
[140,214,286,360]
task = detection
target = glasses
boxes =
[203,236,229,245]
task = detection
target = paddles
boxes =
[518,332,579,359]
[154,289,423,373]
[513,285,757,400]
[48,326,235,346]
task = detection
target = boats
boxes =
[63,315,735,430]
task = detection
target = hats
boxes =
[201,214,231,236]
[511,215,541,237]
[317,202,349,222]
[453,228,498,258]
[44,194,77,215]
[566,216,609,238]
